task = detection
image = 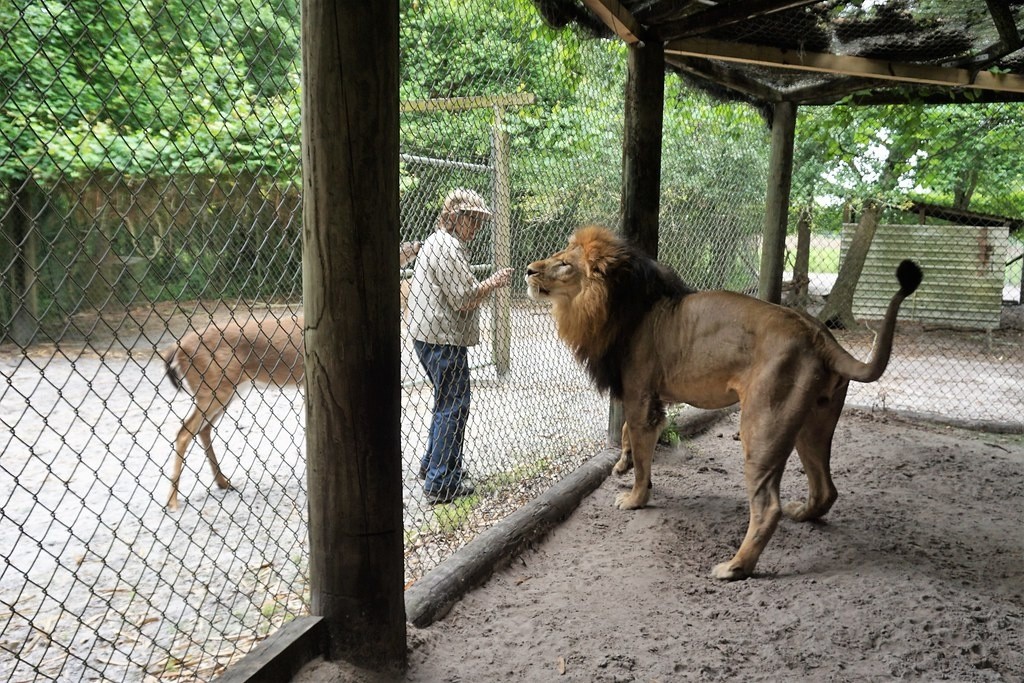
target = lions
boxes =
[525,226,922,581]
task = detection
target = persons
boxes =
[406,187,515,501]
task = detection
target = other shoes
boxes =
[417,458,468,481]
[421,478,475,502]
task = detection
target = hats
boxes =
[441,187,494,217]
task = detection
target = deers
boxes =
[165,241,424,510]
